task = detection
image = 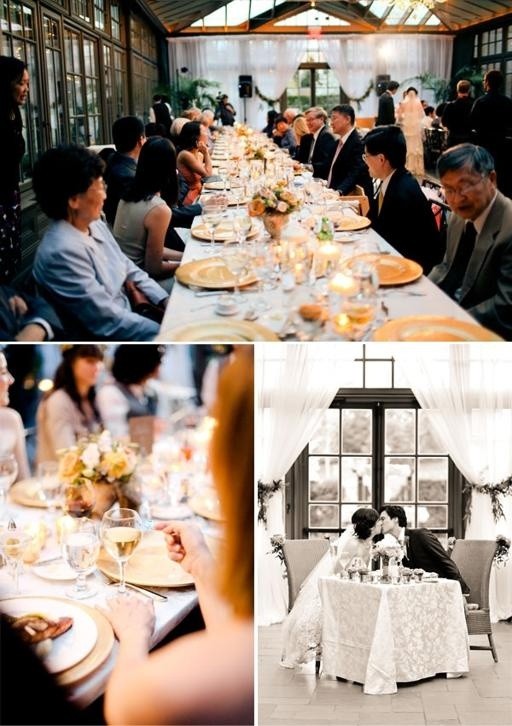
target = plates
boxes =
[96,530,195,589]
[344,253,423,285]
[150,320,281,342]
[372,314,505,341]
[204,181,241,190]
[10,473,66,512]
[334,214,372,231]
[0,598,98,674]
[32,557,93,581]
[214,308,240,315]
[1,595,115,685]
[176,256,258,289]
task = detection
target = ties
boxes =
[439,222,477,298]
[378,183,385,211]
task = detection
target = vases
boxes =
[262,214,289,240]
[78,482,118,520]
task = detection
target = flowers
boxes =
[244,143,268,164]
[266,535,287,578]
[61,430,156,520]
[249,177,299,218]
[495,535,511,569]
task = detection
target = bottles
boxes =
[202,205,222,247]
[371,544,380,572]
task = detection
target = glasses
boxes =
[306,117,321,122]
[82,180,108,191]
[363,152,376,160]
[440,174,485,194]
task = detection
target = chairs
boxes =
[424,127,447,155]
[273,538,331,674]
[448,540,502,663]
[422,180,441,192]
[428,199,452,234]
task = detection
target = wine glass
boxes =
[35,461,63,511]
[0,452,19,505]
[230,178,244,209]
[98,507,143,591]
[328,268,380,341]
[301,164,314,180]
[231,215,252,241]
[310,178,323,201]
[0,532,34,591]
[339,551,351,570]
[60,518,101,599]
[221,240,251,304]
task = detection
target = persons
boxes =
[31,142,170,341]
[379,505,472,618]
[0,285,66,341]
[420,100,428,109]
[395,87,433,177]
[283,109,310,146]
[194,115,213,154]
[169,117,192,136]
[145,123,167,138]
[180,107,201,122]
[176,122,212,208]
[273,118,297,150]
[292,107,335,180]
[360,126,442,276]
[0,351,30,485]
[439,80,476,148]
[292,116,312,162]
[421,106,435,126]
[471,71,512,162]
[262,111,283,138]
[112,136,183,293]
[375,80,399,126]
[150,95,173,129]
[95,344,254,725]
[426,143,512,342]
[198,343,233,411]
[279,508,383,668]
[35,344,103,472]
[326,105,372,197]
[0,54,29,283]
[200,109,215,125]
[104,116,229,253]
[215,94,236,126]
[94,344,171,442]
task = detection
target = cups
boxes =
[216,296,236,311]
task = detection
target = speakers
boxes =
[238,73,253,100]
